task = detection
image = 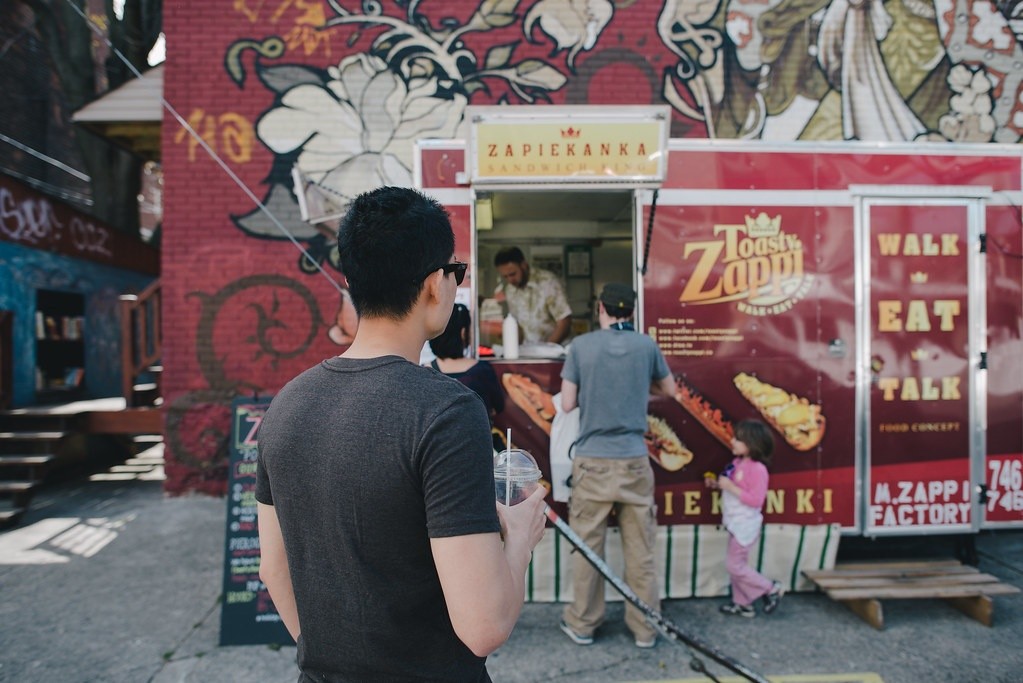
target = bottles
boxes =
[501,314,520,360]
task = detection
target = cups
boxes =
[493,449,542,542]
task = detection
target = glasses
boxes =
[413,261,468,285]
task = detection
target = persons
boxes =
[420,304,505,417]
[558,283,675,647]
[493,246,573,346]
[254,186,547,683]
[704,419,785,618]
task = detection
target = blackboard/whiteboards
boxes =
[220,396,299,646]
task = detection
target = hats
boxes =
[601,283,636,307]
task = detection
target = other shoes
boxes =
[762,580,786,614]
[635,639,656,648]
[559,619,593,644]
[719,602,756,618]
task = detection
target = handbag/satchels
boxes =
[550,392,581,502]
